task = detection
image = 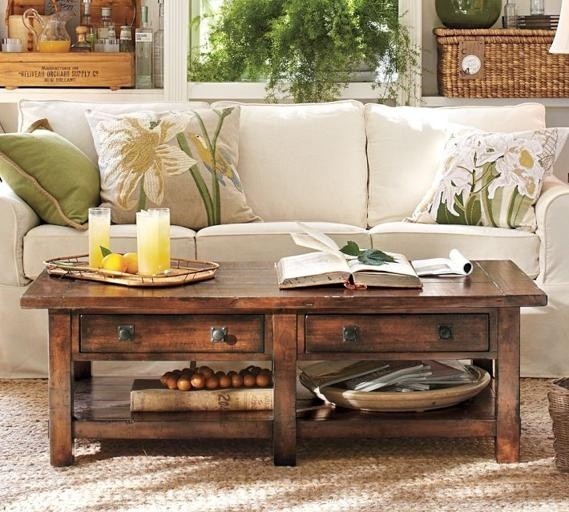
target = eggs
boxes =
[161,366,271,391]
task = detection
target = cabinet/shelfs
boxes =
[0,51,136,91]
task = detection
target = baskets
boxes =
[546,377,569,472]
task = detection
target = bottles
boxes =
[504,0,517,29]
[135,3,164,89]
[70,0,134,52]
[2,38,23,52]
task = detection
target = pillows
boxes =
[0,118,95,231]
[82,102,262,226]
[409,127,568,231]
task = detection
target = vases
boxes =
[434,0,503,29]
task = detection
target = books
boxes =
[274,251,425,292]
[296,358,477,396]
[408,248,474,278]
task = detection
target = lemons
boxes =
[123,252,138,274]
[98,252,127,273]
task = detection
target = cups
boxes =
[136,208,171,276]
[88,207,110,268]
[530,0,544,15]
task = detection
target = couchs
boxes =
[0,97,569,379]
